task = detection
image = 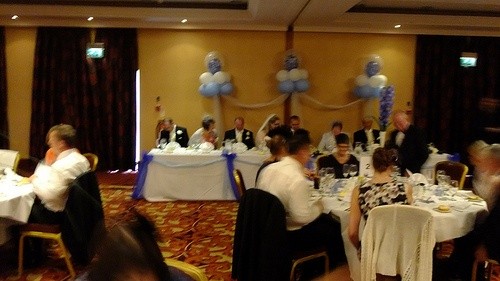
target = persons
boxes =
[188,115,220,150]
[222,116,255,150]
[158,116,189,148]
[316,121,351,155]
[347,146,413,264]
[248,129,348,281]
[283,115,301,137]
[440,140,500,281]
[7,123,92,267]
[254,128,287,189]
[255,113,281,146]
[384,109,429,174]
[352,116,380,152]
[85,211,174,281]
[315,133,360,178]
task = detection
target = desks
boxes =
[132,147,271,202]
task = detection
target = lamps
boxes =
[460,38,478,67]
[86,31,104,58]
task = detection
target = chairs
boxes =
[0,149,97,278]
[227,161,468,281]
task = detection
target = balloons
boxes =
[275,54,309,93]
[354,60,388,98]
[197,58,234,97]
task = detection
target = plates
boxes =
[431,196,462,205]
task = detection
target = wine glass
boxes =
[350,165,358,179]
[342,164,350,180]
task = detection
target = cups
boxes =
[319,168,335,180]
[160,139,167,148]
[437,170,459,196]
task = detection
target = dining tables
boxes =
[0,174,36,244]
[318,173,489,243]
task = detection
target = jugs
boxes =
[221,139,235,151]
